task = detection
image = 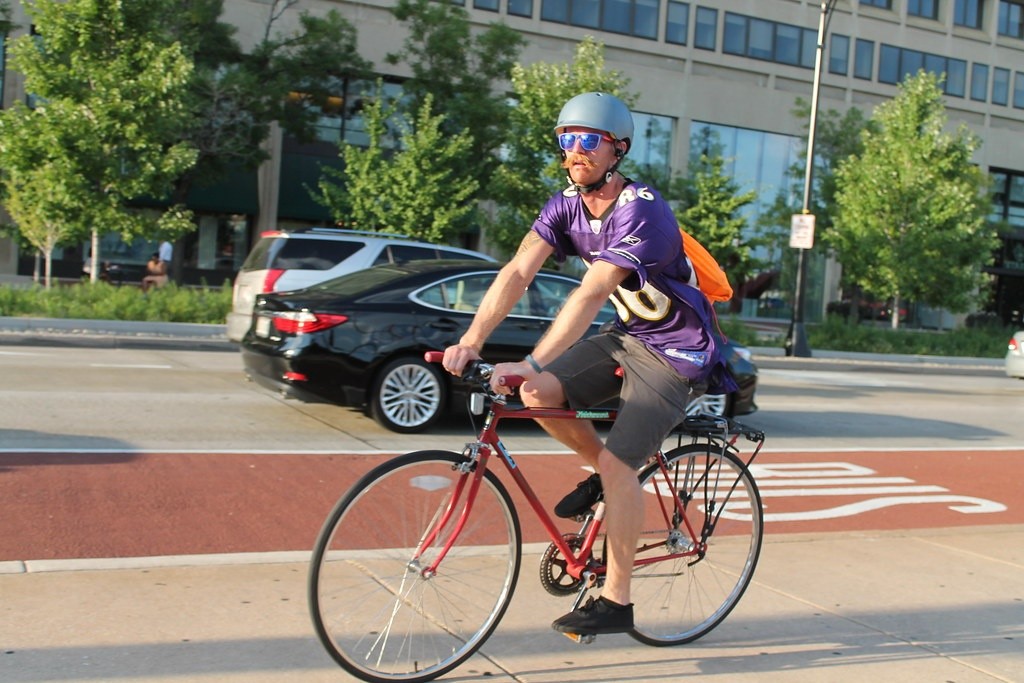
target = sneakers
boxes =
[551,594,634,635]
[553,472,604,519]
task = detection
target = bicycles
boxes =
[304,350,767,683]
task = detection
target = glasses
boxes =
[558,133,614,151]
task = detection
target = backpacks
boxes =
[669,228,734,345]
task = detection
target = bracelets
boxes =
[525,354,543,373]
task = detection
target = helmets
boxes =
[553,91,633,145]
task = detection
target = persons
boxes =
[441,92,733,635]
[145,236,173,275]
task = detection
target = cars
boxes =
[240,259,760,435]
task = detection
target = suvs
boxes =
[221,229,564,344]
